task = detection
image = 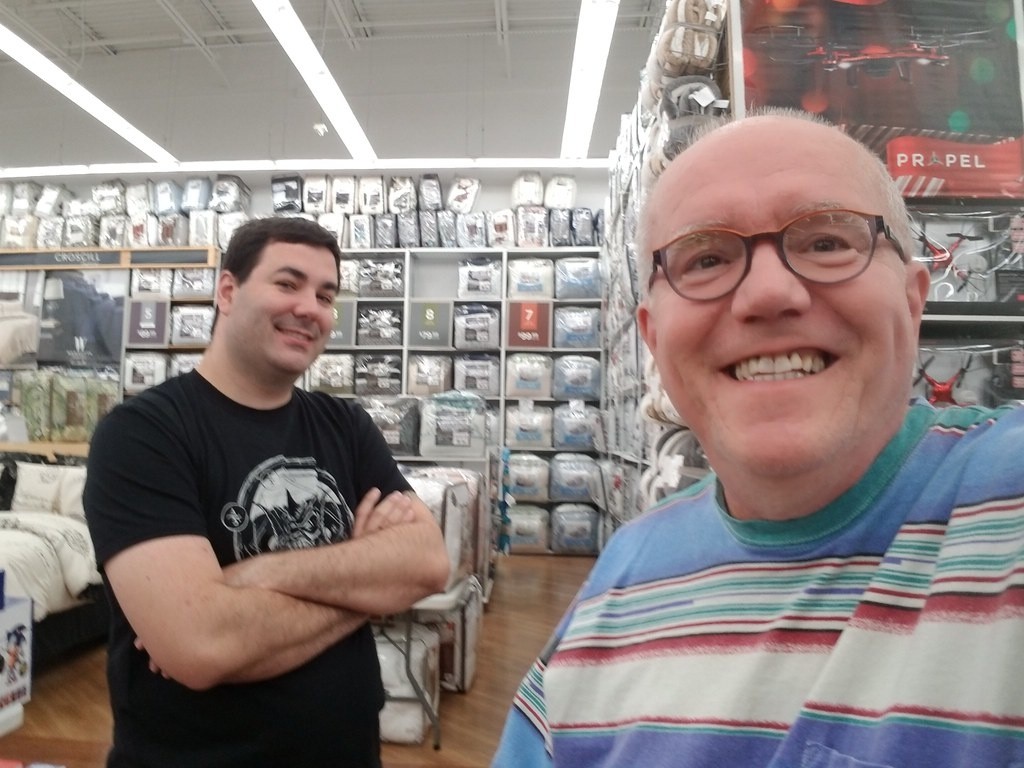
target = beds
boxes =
[0,511,110,682]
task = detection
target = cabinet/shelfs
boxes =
[0,0,1024,604]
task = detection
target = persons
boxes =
[486,106,1024,767]
[81,218,452,767]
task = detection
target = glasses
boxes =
[648,208,908,301]
